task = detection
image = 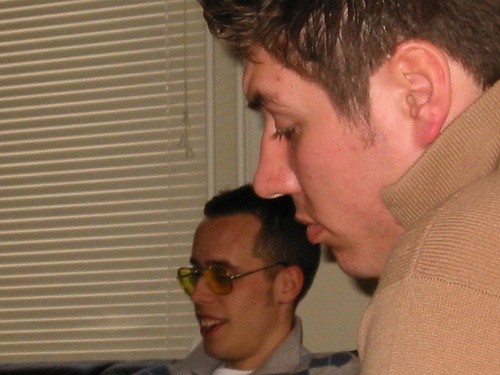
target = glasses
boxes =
[177,262,289,296]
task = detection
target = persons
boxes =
[167,184,360,375]
[194,0,499,375]
